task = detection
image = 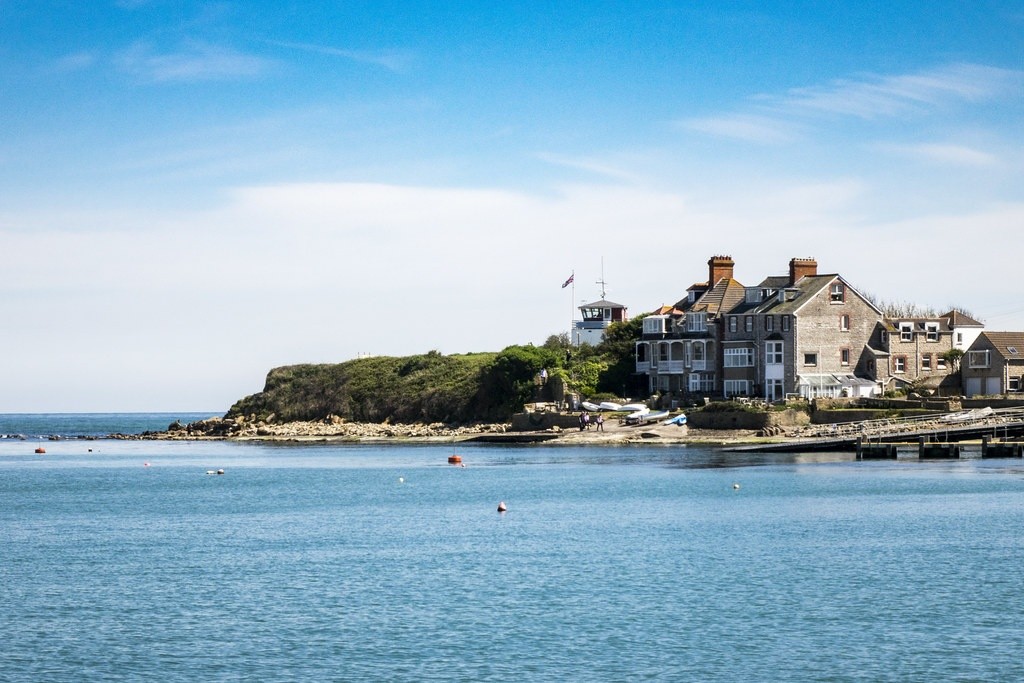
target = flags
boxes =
[562,274,574,288]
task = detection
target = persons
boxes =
[842,389,848,397]
[541,367,547,384]
[566,349,571,361]
[187,423,193,436]
[596,413,604,432]
[578,411,592,431]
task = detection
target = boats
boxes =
[581,401,601,412]
[448,455,463,462]
[625,408,650,420]
[620,403,647,411]
[599,401,621,411]
[664,414,687,425]
[641,409,670,423]
